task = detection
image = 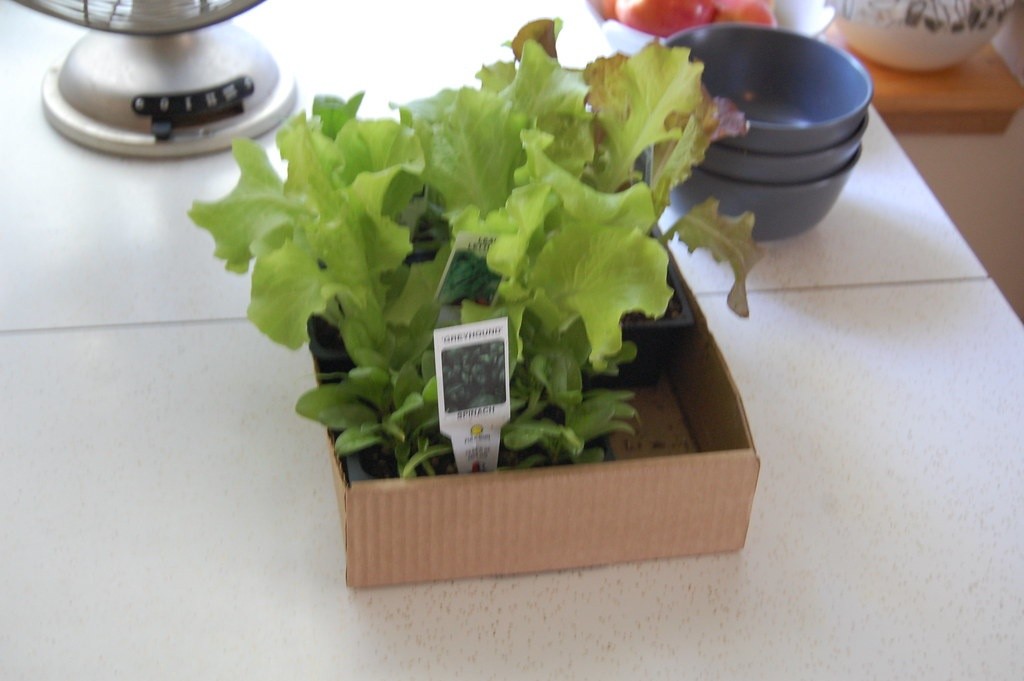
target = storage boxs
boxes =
[310,247,766,589]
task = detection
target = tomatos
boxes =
[596,0,776,38]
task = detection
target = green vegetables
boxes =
[186,16,759,478]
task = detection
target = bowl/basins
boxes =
[834,0,1020,74]
[662,22,875,154]
[686,114,868,184]
[669,142,864,242]
[586,0,835,56]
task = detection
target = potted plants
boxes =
[180,16,767,496]
